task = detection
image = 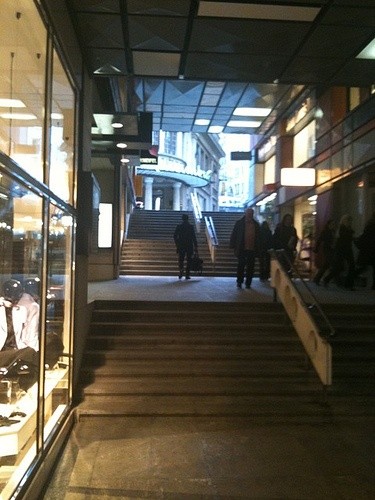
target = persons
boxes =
[312,214,375,291]
[260,221,272,283]
[272,213,297,277]
[0,279,23,353]
[11,279,40,390]
[229,208,261,290]
[173,214,199,279]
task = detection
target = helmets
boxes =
[24,278,41,294]
[2,280,23,301]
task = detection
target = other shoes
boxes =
[323,279,327,287]
[246,285,250,289]
[316,281,320,285]
[237,284,242,289]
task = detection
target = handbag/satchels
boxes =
[190,252,203,271]
